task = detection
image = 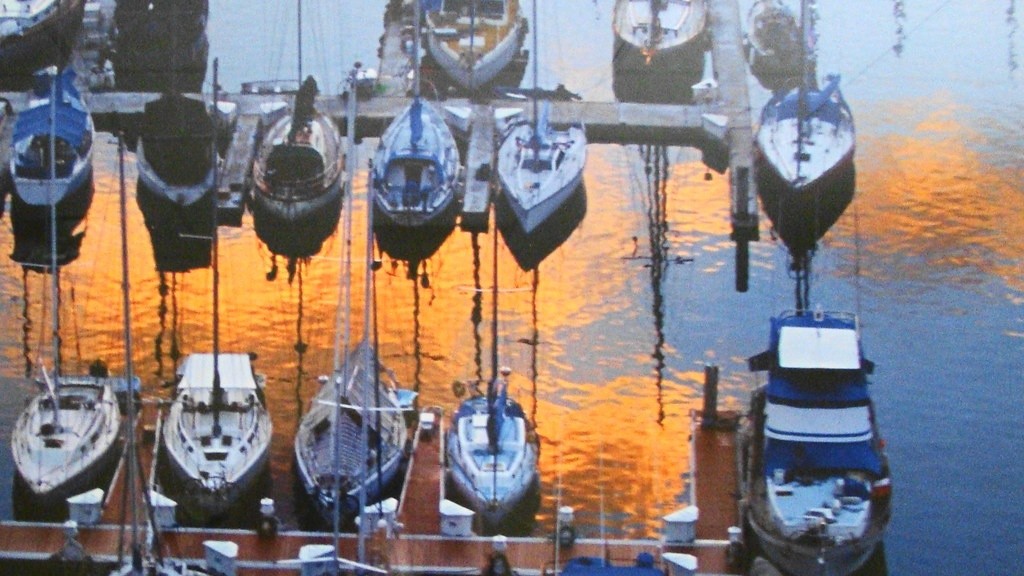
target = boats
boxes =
[1,0,893,576]
